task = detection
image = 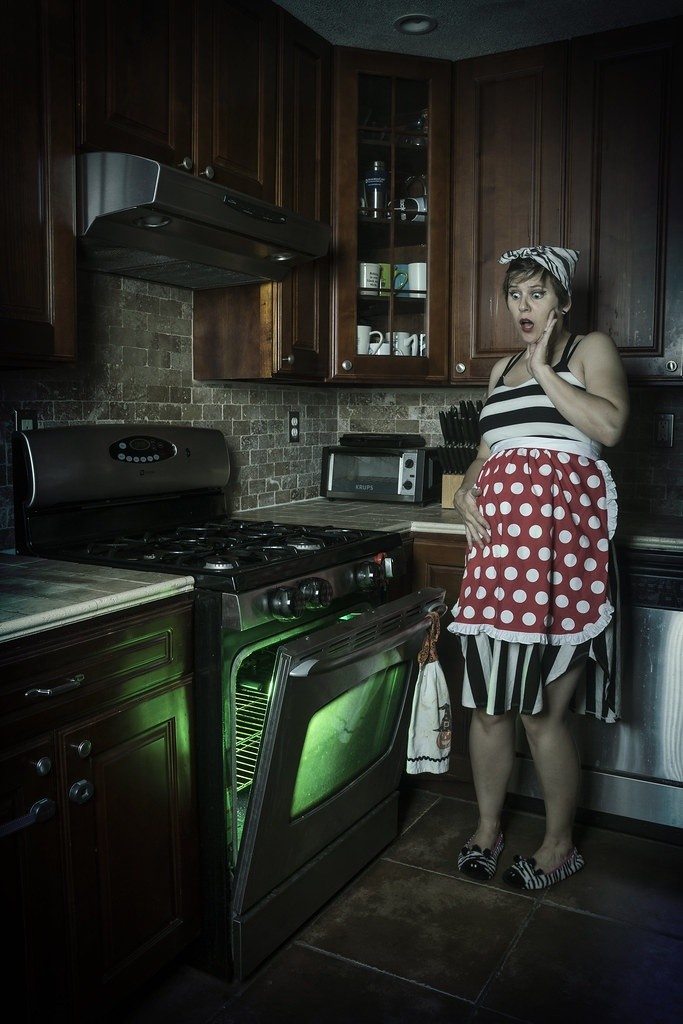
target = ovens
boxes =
[192,539,447,984]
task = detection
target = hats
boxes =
[499,244,581,292]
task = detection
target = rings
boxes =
[543,331,547,334]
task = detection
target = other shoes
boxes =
[457,828,505,881]
[504,844,585,891]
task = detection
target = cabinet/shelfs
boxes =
[0,590,197,1024]
[412,532,473,783]
[0,0,80,368]
[194,5,333,385]
[329,44,452,386]
[74,0,277,204]
[451,15,683,387]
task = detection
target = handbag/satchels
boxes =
[405,608,453,776]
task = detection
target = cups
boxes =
[358,326,427,356]
[359,263,429,298]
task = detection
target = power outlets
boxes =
[15,410,38,431]
[288,411,300,442]
[652,414,674,448]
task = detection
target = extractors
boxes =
[74,154,330,291]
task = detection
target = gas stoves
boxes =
[13,425,403,593]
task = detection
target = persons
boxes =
[446,245,630,890]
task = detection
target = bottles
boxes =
[364,160,389,218]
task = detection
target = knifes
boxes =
[436,400,483,475]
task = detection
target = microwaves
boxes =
[320,445,441,507]
